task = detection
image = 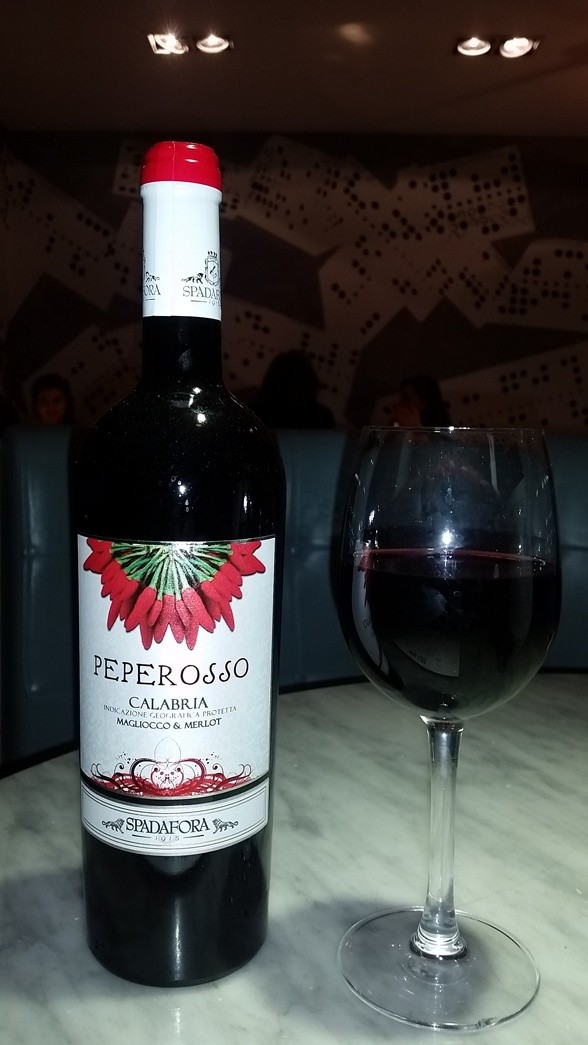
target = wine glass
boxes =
[326,420,563,1030]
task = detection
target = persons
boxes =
[31,373,74,427]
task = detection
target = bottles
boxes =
[67,141,289,992]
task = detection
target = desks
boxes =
[0,676,588,1043]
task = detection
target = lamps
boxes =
[152,25,235,56]
[449,29,541,62]
